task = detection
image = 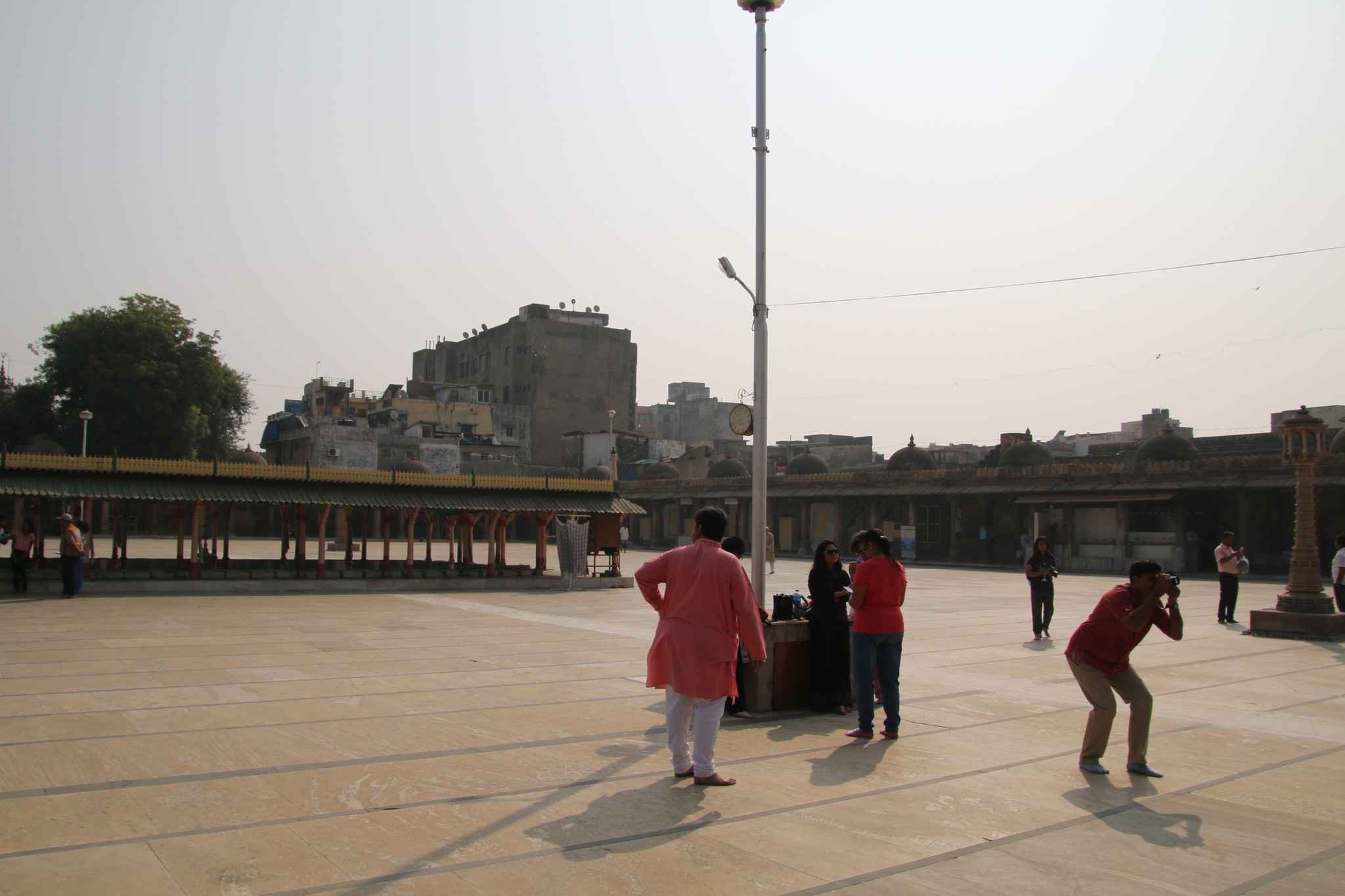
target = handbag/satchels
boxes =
[771,593,810,620]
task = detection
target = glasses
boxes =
[823,549,839,555]
[855,548,866,555]
[737,554,743,561]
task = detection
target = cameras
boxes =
[1163,574,1180,588]
[1047,566,1058,578]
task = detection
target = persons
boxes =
[766,526,777,574]
[808,539,854,715]
[1065,559,1183,778]
[634,505,767,786]
[620,523,629,553]
[1044,523,1058,556]
[845,529,907,739]
[720,537,754,718]
[56,513,95,599]
[1331,534,1345,612]
[1026,536,1058,640]
[0,514,49,594]
[1214,530,1244,624]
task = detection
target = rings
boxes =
[761,660,765,662]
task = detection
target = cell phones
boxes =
[849,561,858,568]
[843,586,853,593]
[1239,547,1244,552]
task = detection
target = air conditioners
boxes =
[327,447,341,457]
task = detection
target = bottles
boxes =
[792,590,801,619]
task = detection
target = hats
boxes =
[56,513,72,521]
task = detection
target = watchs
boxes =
[1167,602,1178,611]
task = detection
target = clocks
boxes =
[729,389,756,436]
